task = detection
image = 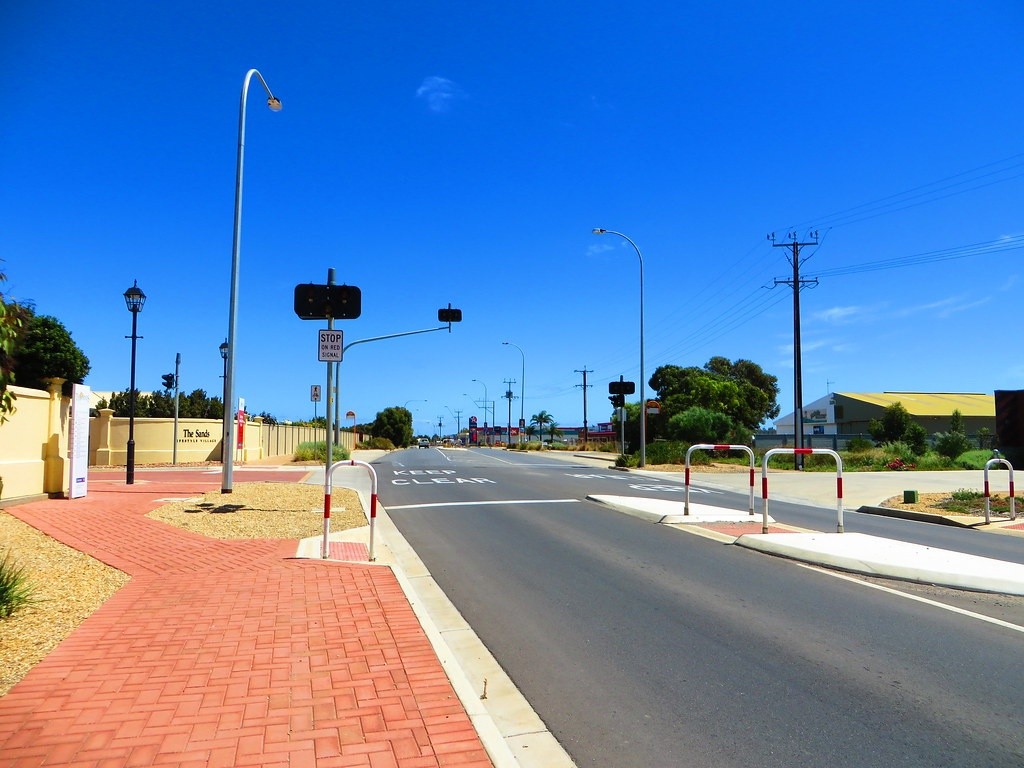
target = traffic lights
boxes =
[294,284,361,320]
[608,396,622,408]
[162,375,174,389]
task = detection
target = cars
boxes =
[419,438,430,449]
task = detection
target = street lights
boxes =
[592,228,647,468]
[444,406,459,439]
[502,342,524,442]
[220,68,282,492]
[219,338,229,462]
[403,399,427,407]
[123,280,145,484]
[463,393,495,447]
[472,379,486,446]
